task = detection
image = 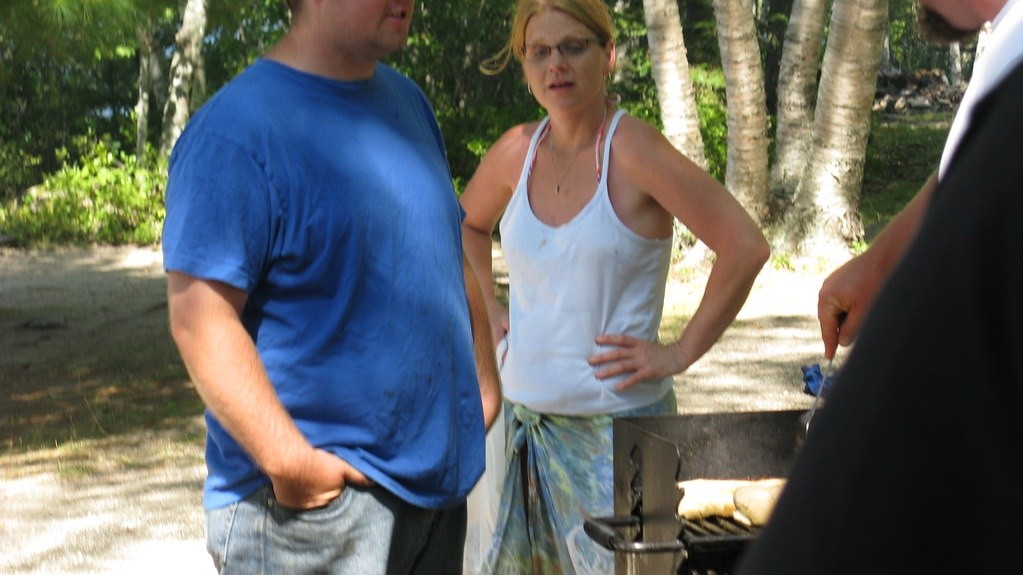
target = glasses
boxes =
[521,38,607,61]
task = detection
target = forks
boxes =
[793,358,834,449]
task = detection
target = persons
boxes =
[736,0,1023,575]
[161,0,503,575]
[459,0,770,575]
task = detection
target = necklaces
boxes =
[550,103,604,194]
